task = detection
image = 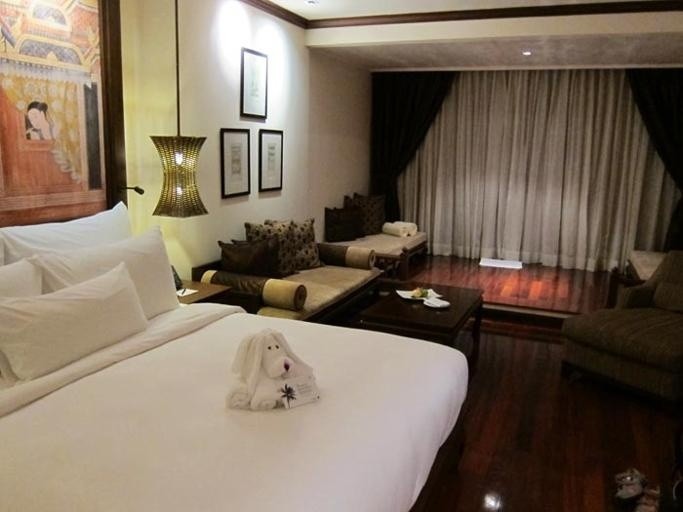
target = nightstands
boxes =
[171,273,230,304]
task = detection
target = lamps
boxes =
[147,0,209,219]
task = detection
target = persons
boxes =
[25,101,55,139]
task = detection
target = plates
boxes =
[394,288,443,301]
[422,299,450,308]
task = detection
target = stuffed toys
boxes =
[221,325,317,411]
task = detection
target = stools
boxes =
[619,244,665,285]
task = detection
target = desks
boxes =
[357,280,483,355]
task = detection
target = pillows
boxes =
[0,250,46,297]
[243,217,298,281]
[231,233,281,280]
[216,238,271,278]
[34,224,180,325]
[263,215,324,271]
[0,201,136,257]
[0,200,473,512]
[315,242,375,269]
[201,269,307,312]
[1,258,147,385]
[321,192,388,242]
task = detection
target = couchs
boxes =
[550,245,682,402]
[325,219,430,282]
[188,239,391,322]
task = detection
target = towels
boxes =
[382,219,417,238]
[479,250,527,271]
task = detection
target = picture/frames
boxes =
[256,125,285,192]
[0,0,128,220]
[238,42,269,121]
[219,126,252,199]
